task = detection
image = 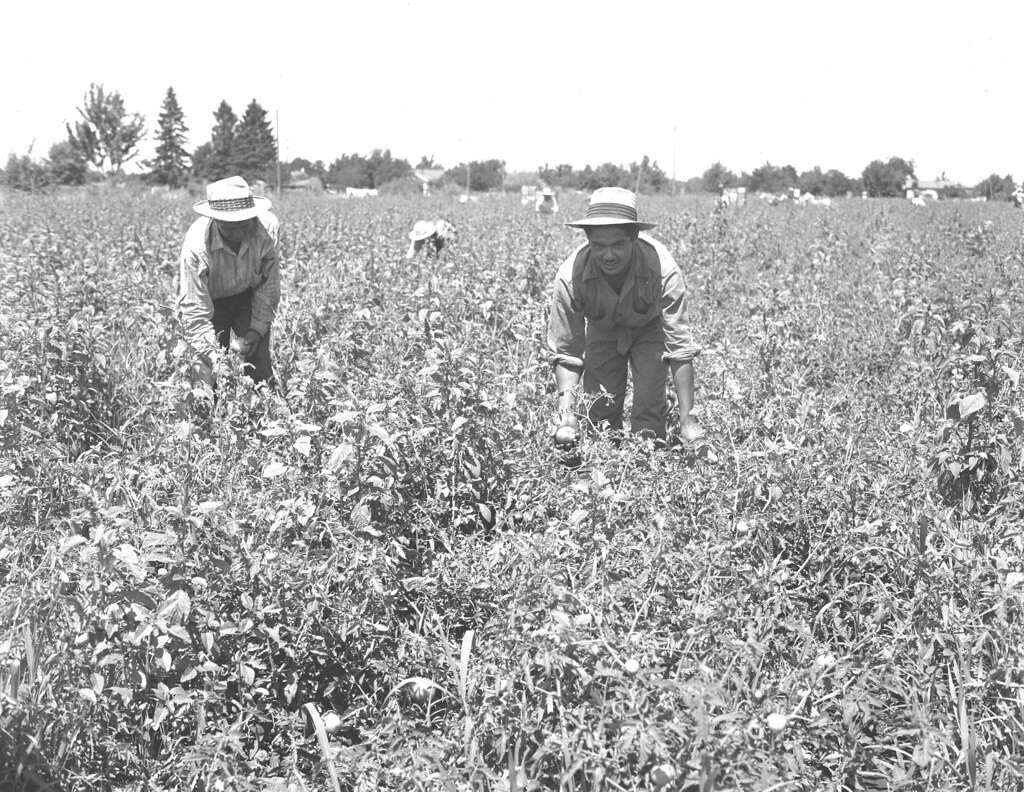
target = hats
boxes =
[193,175,272,223]
[565,188,658,230]
[541,188,553,196]
[407,220,435,242]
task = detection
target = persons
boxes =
[536,187,558,215]
[1011,185,1024,209]
[548,185,705,451]
[176,175,285,398]
[407,218,446,263]
